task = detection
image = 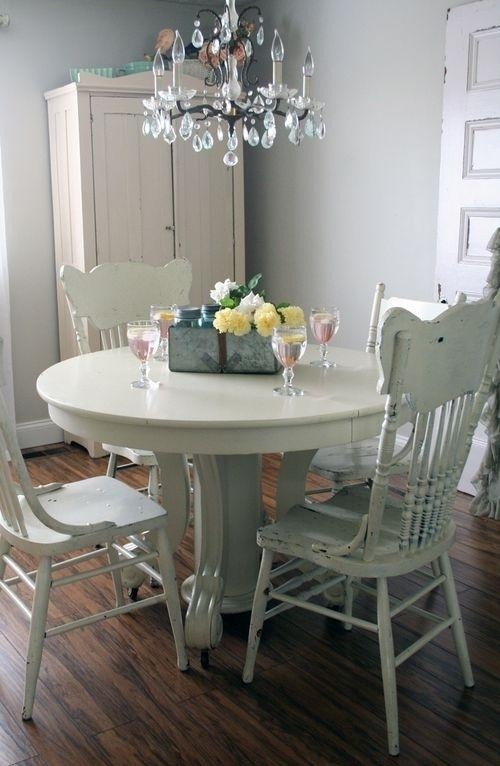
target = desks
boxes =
[32,341,418,671]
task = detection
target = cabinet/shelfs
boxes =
[43,68,246,463]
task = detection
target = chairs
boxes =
[280,283,466,497]
[0,339,189,721]
[242,288,498,754]
[60,258,194,504]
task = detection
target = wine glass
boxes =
[271,306,339,398]
[126,304,178,390]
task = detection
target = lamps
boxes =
[136,0,326,170]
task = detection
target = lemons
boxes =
[159,312,174,319]
[283,334,304,344]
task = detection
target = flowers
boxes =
[207,273,304,338]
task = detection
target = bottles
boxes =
[175,304,225,327]
